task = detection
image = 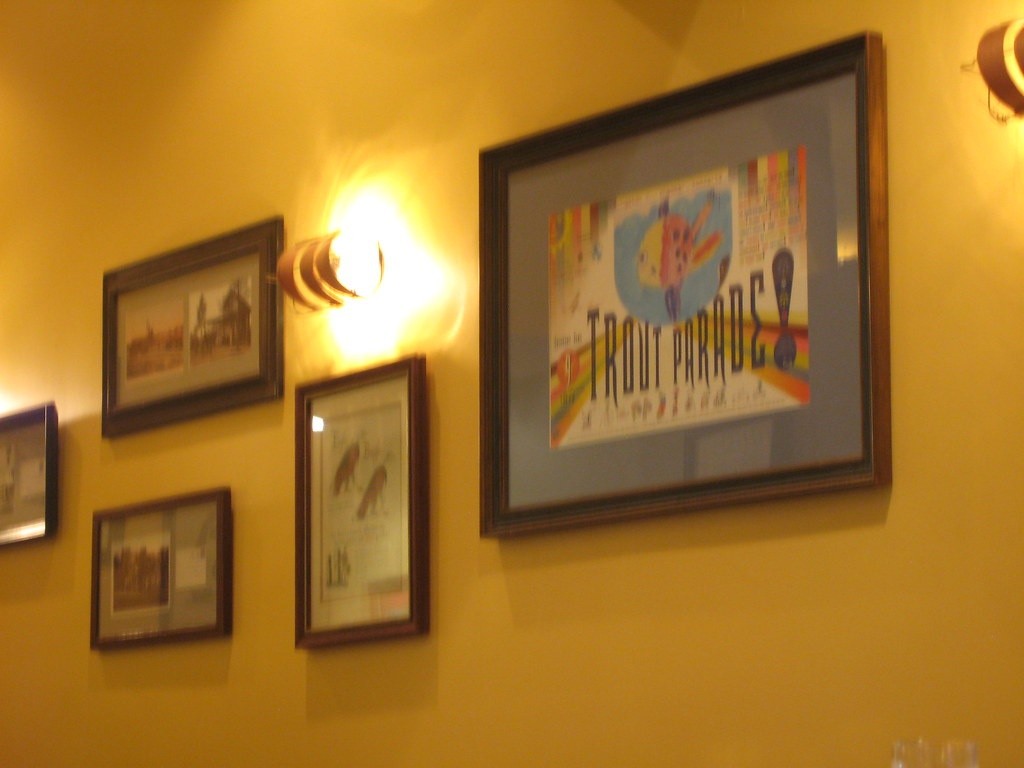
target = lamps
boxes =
[977,19,1024,122]
[277,231,383,310]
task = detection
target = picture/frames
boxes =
[90,486,232,648]
[478,31,893,541]
[295,352,426,649]
[0,400,58,545]
[99,216,283,438]
